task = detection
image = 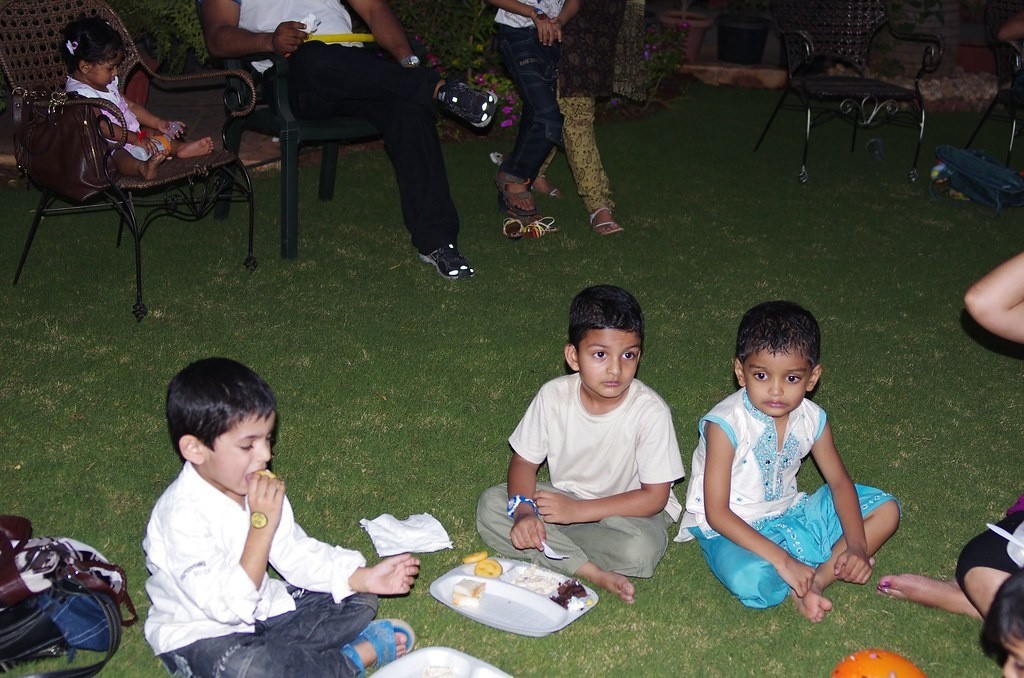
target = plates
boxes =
[431,556,599,636]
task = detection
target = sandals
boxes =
[341,618,416,678]
[590,206,623,235]
[495,168,537,216]
[532,174,561,198]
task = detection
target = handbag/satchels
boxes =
[0,515,139,678]
[18,89,109,206]
[929,144,1024,219]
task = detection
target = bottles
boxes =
[131,136,172,162]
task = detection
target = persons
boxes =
[877,251,1024,621]
[143,357,420,678]
[998,12,1024,135]
[673,300,900,624]
[489,0,579,219]
[530,0,648,234]
[477,285,687,604]
[197,0,498,282]
[991,568,1024,678]
[58,16,215,182]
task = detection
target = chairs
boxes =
[963,0,1024,166]
[0,0,258,322]
[749,0,947,184]
[195,0,385,260]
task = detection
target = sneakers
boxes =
[435,76,498,128]
[420,242,475,280]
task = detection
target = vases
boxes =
[660,10,710,63]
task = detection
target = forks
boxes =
[541,541,570,559]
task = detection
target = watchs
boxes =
[401,55,420,66]
[506,495,539,518]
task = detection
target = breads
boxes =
[462,551,503,578]
[453,580,487,605]
[255,471,281,490]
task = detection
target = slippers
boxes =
[502,214,560,240]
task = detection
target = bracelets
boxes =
[552,17,564,28]
